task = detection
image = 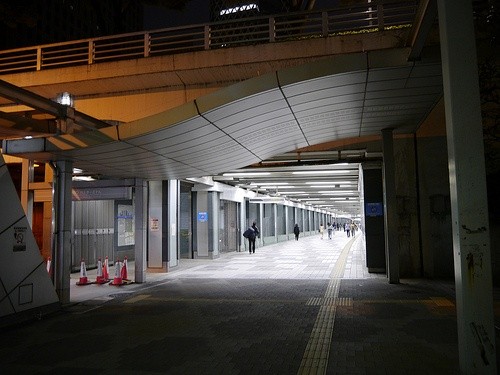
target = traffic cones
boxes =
[121,256,132,281]
[76,259,93,285]
[109,259,128,286]
[47,256,52,274]
[94,257,113,284]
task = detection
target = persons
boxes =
[248,222,260,255]
[319,224,324,239]
[326,220,357,239]
[294,224,299,241]
